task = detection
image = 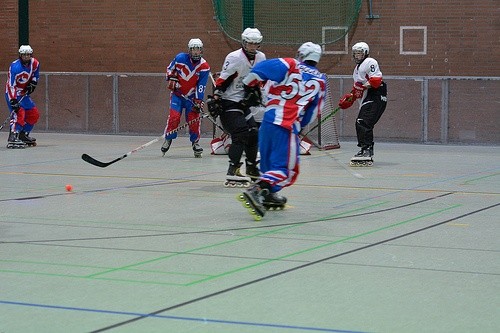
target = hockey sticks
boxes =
[174,86,228,136]
[300,97,353,141]
[80,111,210,168]
[0,93,28,130]
[256,102,322,150]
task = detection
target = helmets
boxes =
[351,41,369,64]
[295,42,322,63]
[241,27,263,58]
[18,44,33,66]
[187,38,204,61]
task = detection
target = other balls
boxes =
[67,185,72,191]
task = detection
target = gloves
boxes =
[351,81,367,100]
[10,99,20,112]
[24,80,37,96]
[338,93,356,109]
[191,99,205,113]
[166,76,179,92]
[242,84,262,108]
[206,94,224,119]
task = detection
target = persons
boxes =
[237,42,329,221]
[207,28,267,188]
[161,38,210,158]
[5,45,41,149]
[350,41,387,167]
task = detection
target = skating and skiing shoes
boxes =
[245,159,262,183]
[263,192,287,210]
[236,181,272,221]
[191,139,203,158]
[224,161,251,188]
[19,130,37,146]
[7,129,27,149]
[161,138,172,157]
[350,142,375,167]
